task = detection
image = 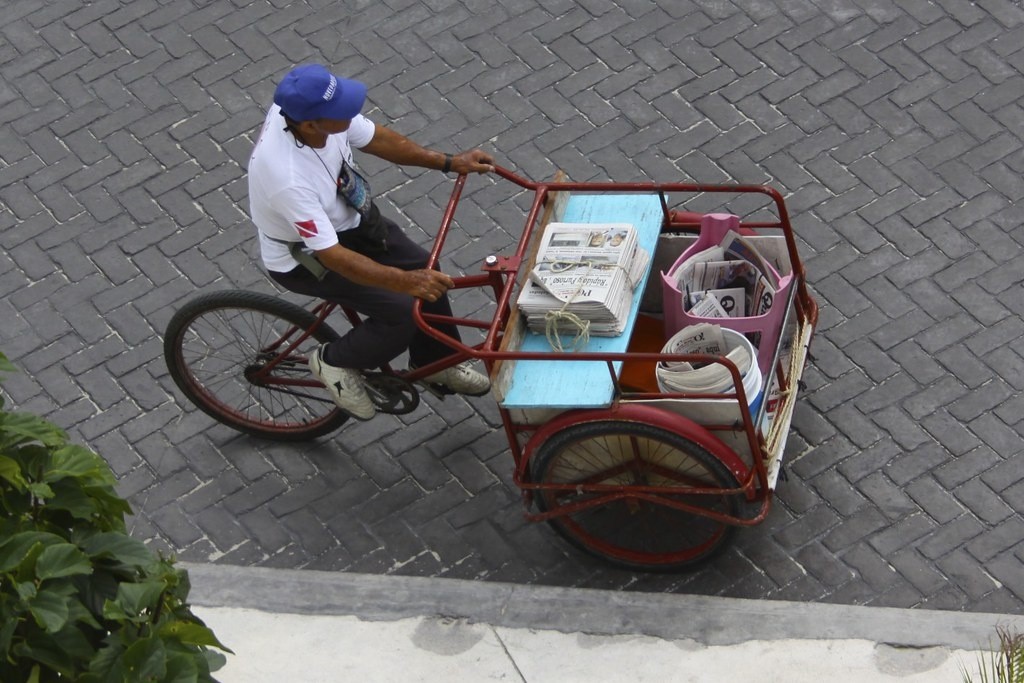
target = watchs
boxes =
[442,153,453,173]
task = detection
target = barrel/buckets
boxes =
[655,327,769,440]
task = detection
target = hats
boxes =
[274,64,367,120]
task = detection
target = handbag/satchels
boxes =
[337,161,371,222]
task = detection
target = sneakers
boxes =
[308,342,377,421]
[408,358,491,396]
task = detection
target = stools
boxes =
[660,213,795,375]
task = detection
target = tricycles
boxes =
[162,154,820,573]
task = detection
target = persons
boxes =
[249,65,489,422]
